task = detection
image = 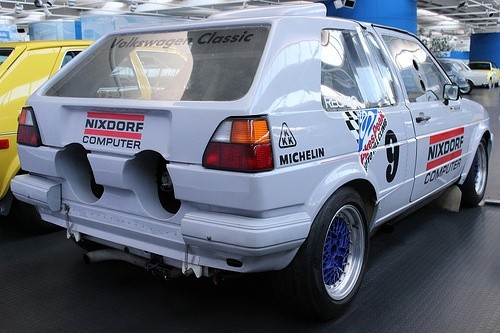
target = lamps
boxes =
[14,4,24,15]
[130,2,138,12]
[68,0,75,6]
[43,5,51,16]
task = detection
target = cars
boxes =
[438,59,488,95]
[0,40,90,227]
[467,61,500,89]
[8,4,496,322]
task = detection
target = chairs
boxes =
[193,57,251,99]
